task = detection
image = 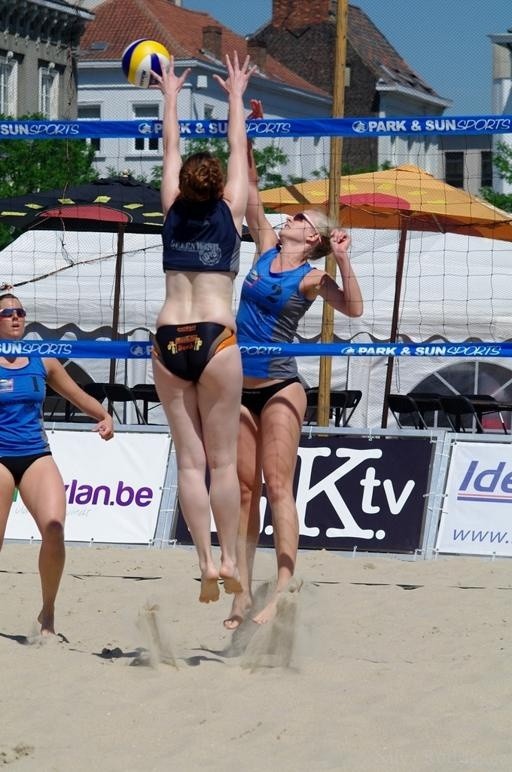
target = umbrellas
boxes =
[0,179,258,415]
[256,163,512,430]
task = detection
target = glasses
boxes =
[294,212,322,246]
[0,308,26,318]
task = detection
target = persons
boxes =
[219,95,364,632]
[1,293,113,641]
[141,49,259,604]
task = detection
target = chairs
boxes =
[34,380,161,432]
[305,379,511,435]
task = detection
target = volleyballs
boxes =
[120,38,172,91]
